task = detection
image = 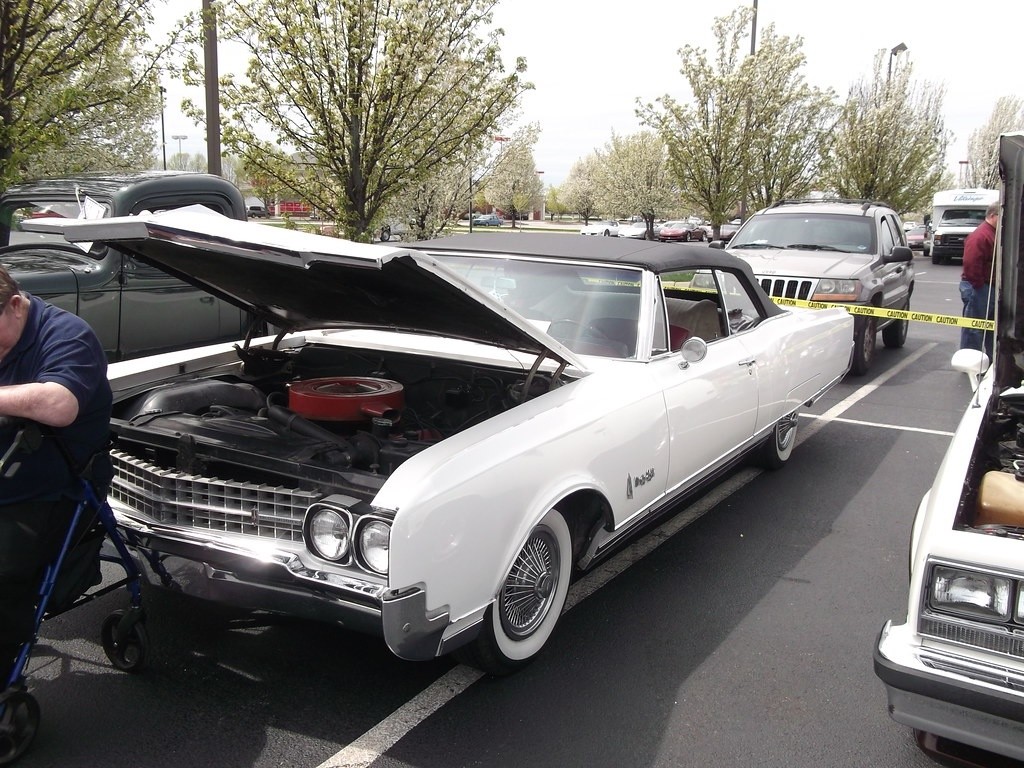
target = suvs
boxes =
[246,205,271,218]
[688,196,915,377]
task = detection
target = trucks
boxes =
[924,189,1000,264]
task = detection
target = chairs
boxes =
[581,317,693,355]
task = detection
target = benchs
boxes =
[530,288,722,341]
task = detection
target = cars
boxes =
[1,171,259,368]
[309,211,320,220]
[579,220,620,237]
[618,222,657,240]
[378,214,416,241]
[872,130,1024,767]
[473,214,503,227]
[903,222,932,257]
[18,204,854,676]
[464,210,483,220]
[652,218,742,243]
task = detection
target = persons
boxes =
[0,265,115,612]
[959,201,1000,376]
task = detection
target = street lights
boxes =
[157,86,167,170]
[884,42,909,98]
[172,134,187,165]
